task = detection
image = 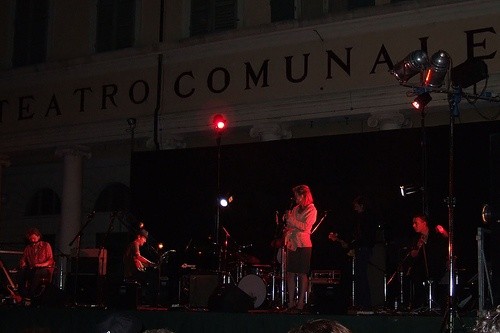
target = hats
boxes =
[138,228,148,238]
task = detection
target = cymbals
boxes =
[253,263,271,268]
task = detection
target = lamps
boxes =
[391,50,451,109]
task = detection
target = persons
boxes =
[14,309,500,333]
[409,213,463,280]
[283,183,318,311]
[121,229,158,279]
[341,194,384,250]
[18,228,57,287]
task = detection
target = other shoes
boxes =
[281,304,305,313]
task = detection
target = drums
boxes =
[235,272,267,308]
[218,270,235,288]
[228,250,245,266]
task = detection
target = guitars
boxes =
[328,232,355,257]
[0,261,22,302]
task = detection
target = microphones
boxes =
[88,209,96,214]
[222,227,230,237]
[324,211,328,224]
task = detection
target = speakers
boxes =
[207,284,252,313]
[190,274,219,309]
